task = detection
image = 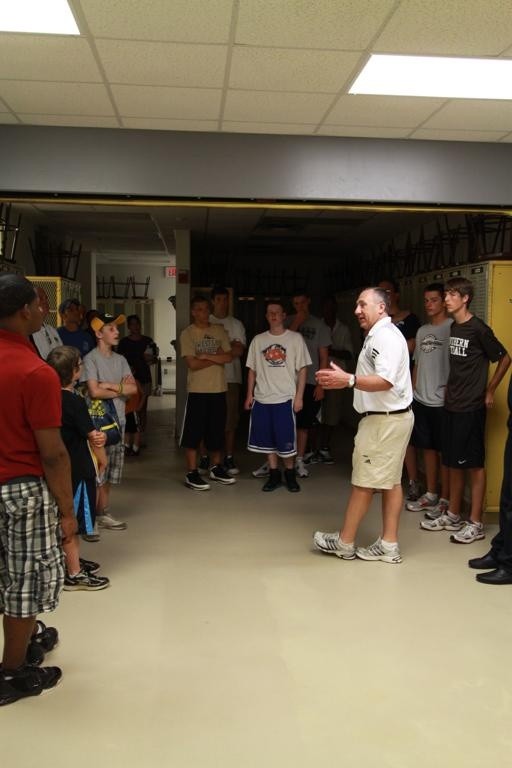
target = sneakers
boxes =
[405,478,485,543]
[355,537,402,563]
[184,447,335,492]
[313,530,356,559]
[95,513,128,530]
[26,620,58,666]
[65,558,101,574]
[83,521,100,542]
[0,663,62,707]
[63,569,110,591]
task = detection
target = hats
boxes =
[88,311,127,334]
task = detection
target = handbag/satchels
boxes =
[75,381,122,446]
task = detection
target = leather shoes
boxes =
[476,566,512,585]
[468,553,497,569]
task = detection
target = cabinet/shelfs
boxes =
[27,275,86,330]
[97,300,157,394]
[400,260,512,524]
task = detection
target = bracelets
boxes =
[349,374,357,388]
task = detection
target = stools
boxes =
[132,276,150,299]
[26,235,84,279]
[0,202,23,266]
[96,274,113,300]
[192,205,512,292]
[112,274,131,298]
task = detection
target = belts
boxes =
[361,405,412,418]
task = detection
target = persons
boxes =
[179,278,455,520]
[313,287,414,563]
[469,382,512,585]
[1,275,159,706]
[420,277,511,543]
